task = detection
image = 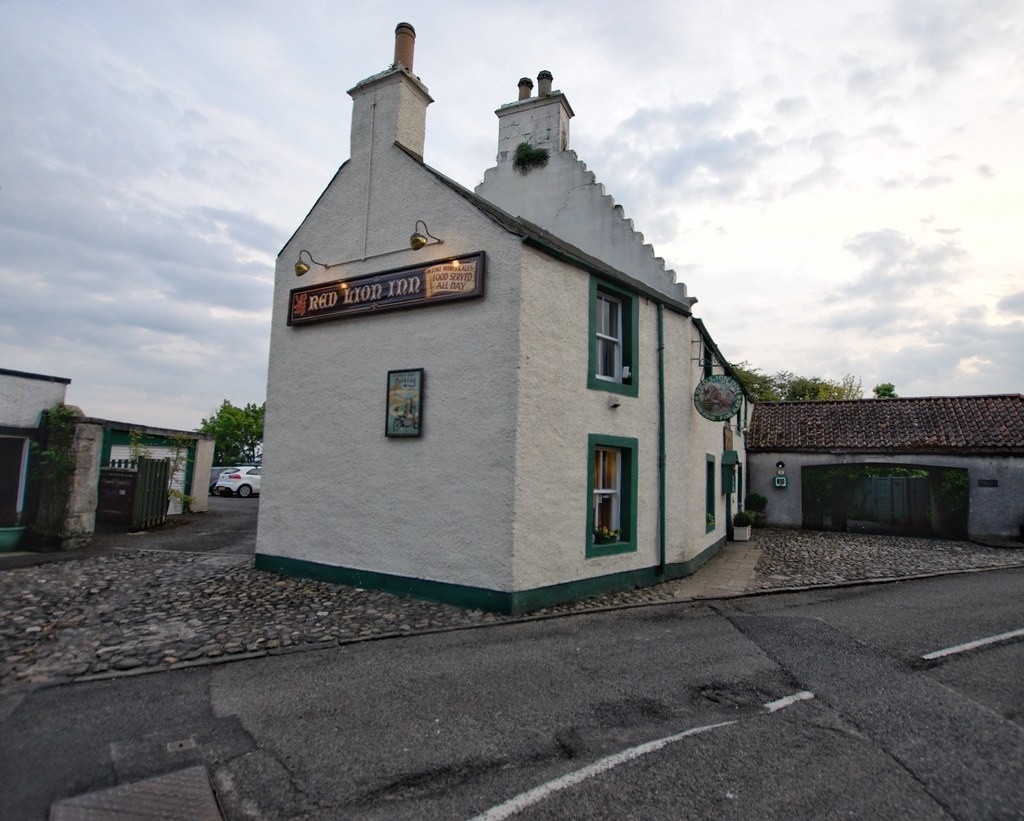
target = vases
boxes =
[594,536,616,545]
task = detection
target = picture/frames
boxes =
[386,367,423,439]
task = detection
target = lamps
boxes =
[728,419,748,432]
[410,220,441,251]
[294,250,328,277]
[776,460,785,469]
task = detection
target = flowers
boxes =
[592,524,623,537]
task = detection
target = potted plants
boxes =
[0,503,27,551]
[733,513,754,541]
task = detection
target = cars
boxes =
[209,465,262,498]
